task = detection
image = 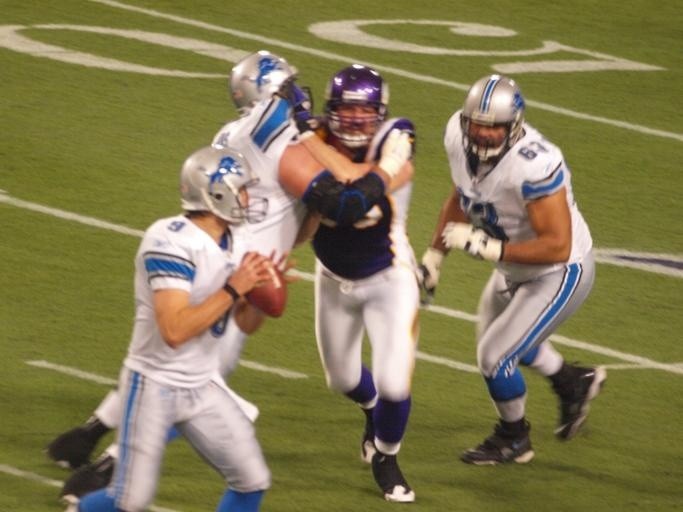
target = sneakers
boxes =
[43,430,95,472]
[59,473,106,507]
[360,415,376,464]
[459,422,535,467]
[370,455,415,503]
[552,363,608,442]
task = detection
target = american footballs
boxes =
[242,251,288,318]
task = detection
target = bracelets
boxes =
[221,282,239,303]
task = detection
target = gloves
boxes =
[441,221,503,263]
[378,128,413,180]
[285,82,315,135]
[416,245,444,304]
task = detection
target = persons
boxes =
[281,64,422,506]
[62,141,303,512]
[42,49,413,509]
[418,73,608,467]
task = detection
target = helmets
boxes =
[179,145,270,226]
[229,50,315,114]
[457,75,527,174]
[320,64,391,152]
